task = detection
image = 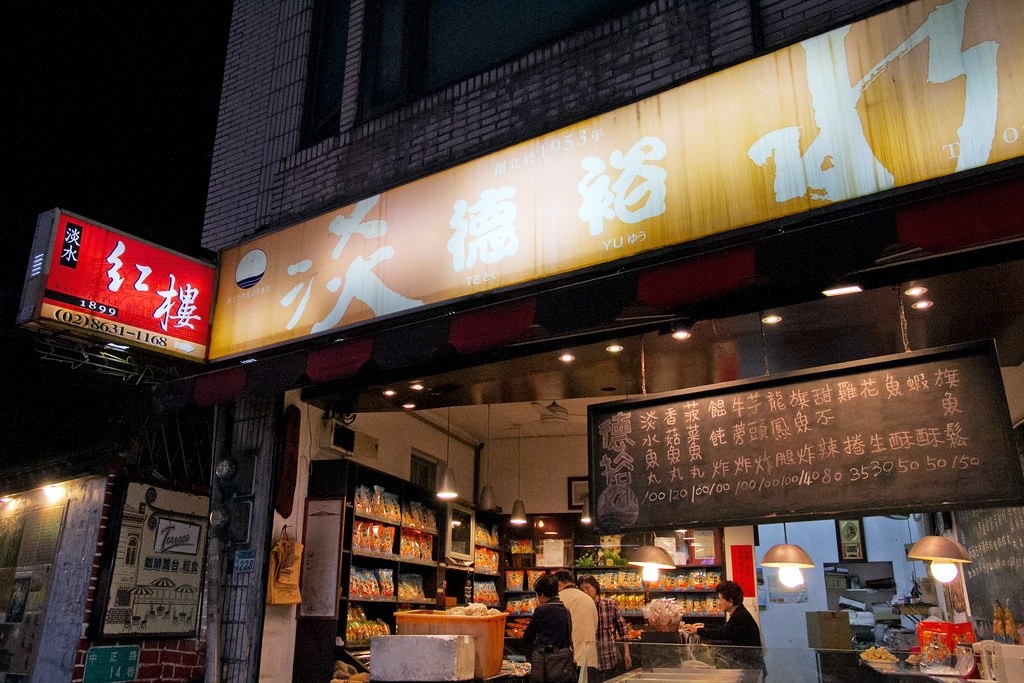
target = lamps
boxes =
[627,527,676,582]
[582,498,593,524]
[435,407,459,496]
[907,508,973,582]
[511,425,526,528]
[761,523,815,587]
[382,282,936,411]
[479,404,496,508]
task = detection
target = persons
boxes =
[554,568,599,683]
[578,575,632,682]
[684,581,767,678]
[523,574,578,683]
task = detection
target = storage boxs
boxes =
[368,635,476,681]
[543,539,568,567]
[392,610,511,679]
[806,609,852,654]
[654,536,676,566]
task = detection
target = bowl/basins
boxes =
[972,642,1024,683]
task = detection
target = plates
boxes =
[860,654,900,663]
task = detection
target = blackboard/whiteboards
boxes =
[950,508,1024,624]
[584,337,1024,536]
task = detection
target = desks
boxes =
[863,660,960,683]
[815,648,919,683]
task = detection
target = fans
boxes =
[505,400,587,430]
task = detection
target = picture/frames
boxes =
[836,518,867,562]
[568,477,589,510]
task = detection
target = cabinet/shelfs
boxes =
[505,520,727,637]
[292,459,502,683]
